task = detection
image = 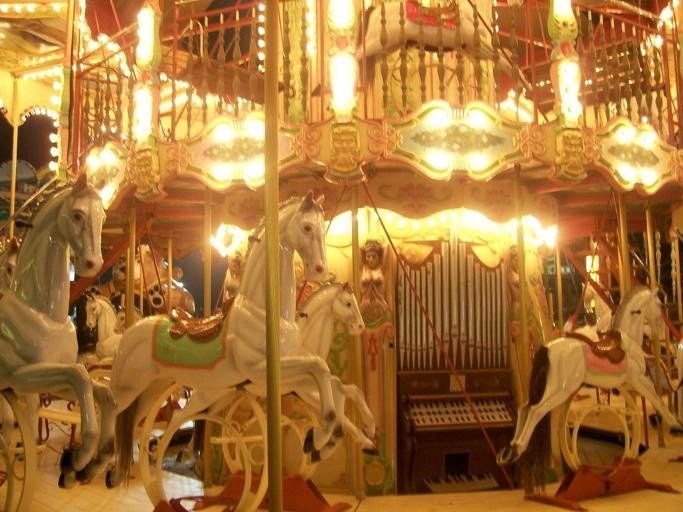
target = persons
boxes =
[357,239,394,348]
[223,248,245,301]
